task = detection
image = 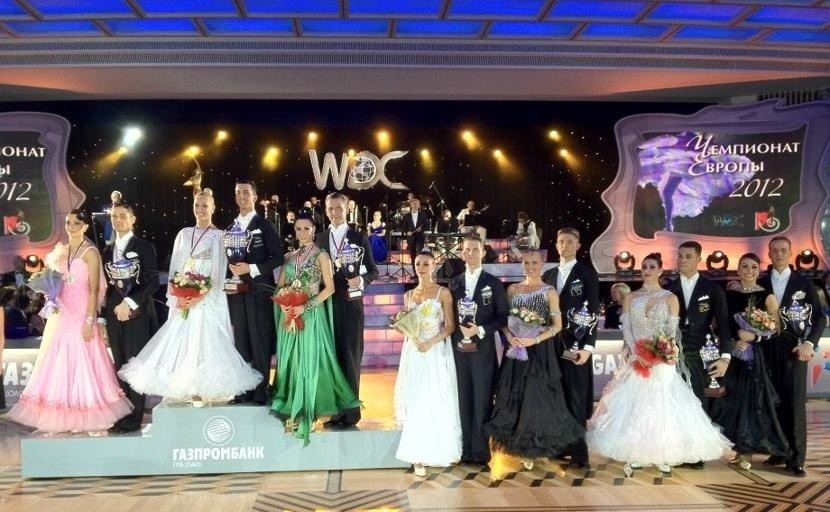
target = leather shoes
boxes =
[323,418,338,427]
[337,415,360,427]
[480,463,490,472]
[228,398,240,404]
[763,455,785,466]
[631,462,643,468]
[119,427,130,433]
[739,460,752,469]
[727,455,741,465]
[108,427,119,432]
[792,466,807,477]
[457,460,466,465]
[693,462,703,470]
[252,389,267,406]
[656,464,670,473]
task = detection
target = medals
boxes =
[66,240,86,282]
[187,225,213,267]
[292,243,315,288]
[330,227,351,267]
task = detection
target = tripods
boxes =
[391,246,413,279]
[377,257,397,282]
[496,242,521,263]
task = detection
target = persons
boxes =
[604,283,630,329]
[270,207,366,448]
[5,256,34,288]
[457,201,486,244]
[482,254,586,470]
[366,211,388,265]
[585,254,736,479]
[511,212,541,263]
[105,191,121,251]
[710,254,789,470]
[395,193,415,217]
[449,237,508,470]
[6,294,38,339]
[542,229,600,468]
[756,237,827,476]
[223,179,286,404]
[388,251,463,477]
[282,211,300,255]
[304,201,324,233]
[402,199,430,281]
[260,195,287,229]
[437,209,456,233]
[96,202,160,434]
[662,241,731,469]
[4,210,134,438]
[117,188,265,409]
[0,288,14,408]
[315,193,379,429]
[311,196,322,215]
[347,201,366,235]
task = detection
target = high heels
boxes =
[524,461,533,470]
[414,464,427,476]
[191,399,203,408]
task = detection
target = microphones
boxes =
[428,180,435,190]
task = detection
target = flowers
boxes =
[26,268,64,318]
[506,306,547,360]
[631,331,679,379]
[270,285,306,334]
[389,307,424,347]
[733,295,780,360]
[168,271,213,319]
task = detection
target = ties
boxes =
[15,271,23,275]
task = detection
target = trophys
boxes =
[779,296,814,359]
[456,289,478,352]
[334,234,365,303]
[561,300,599,361]
[221,218,253,294]
[105,249,140,317]
[699,334,727,397]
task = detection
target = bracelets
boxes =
[85,315,93,320]
